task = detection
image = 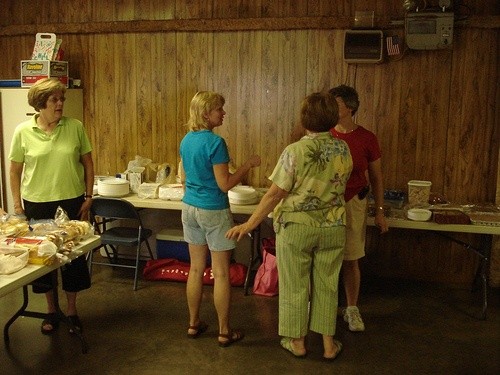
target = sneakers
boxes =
[343,308,365,331]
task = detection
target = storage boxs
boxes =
[21,59,69,89]
[156,227,211,266]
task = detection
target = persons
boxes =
[328,85,388,331]
[225,94,353,358]
[10,78,94,333]
[179,91,261,346]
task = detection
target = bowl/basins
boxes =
[158,184,183,201]
[408,209,431,222]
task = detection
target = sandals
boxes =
[218,330,245,347]
[67,315,82,337]
[41,311,62,333]
[188,324,207,337]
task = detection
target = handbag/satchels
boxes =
[253,236,278,296]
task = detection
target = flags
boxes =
[387,36,400,55]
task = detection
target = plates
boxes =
[97,178,130,198]
[228,186,256,204]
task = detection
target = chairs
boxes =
[91,198,154,293]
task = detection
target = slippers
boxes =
[280,338,305,357]
[323,340,343,360]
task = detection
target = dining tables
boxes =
[0,208,102,375]
[94,182,262,296]
[264,186,500,320]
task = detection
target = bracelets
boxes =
[86,195,92,198]
[376,206,384,213]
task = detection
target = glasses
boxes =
[47,97,66,103]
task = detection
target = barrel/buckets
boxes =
[408,180,432,203]
